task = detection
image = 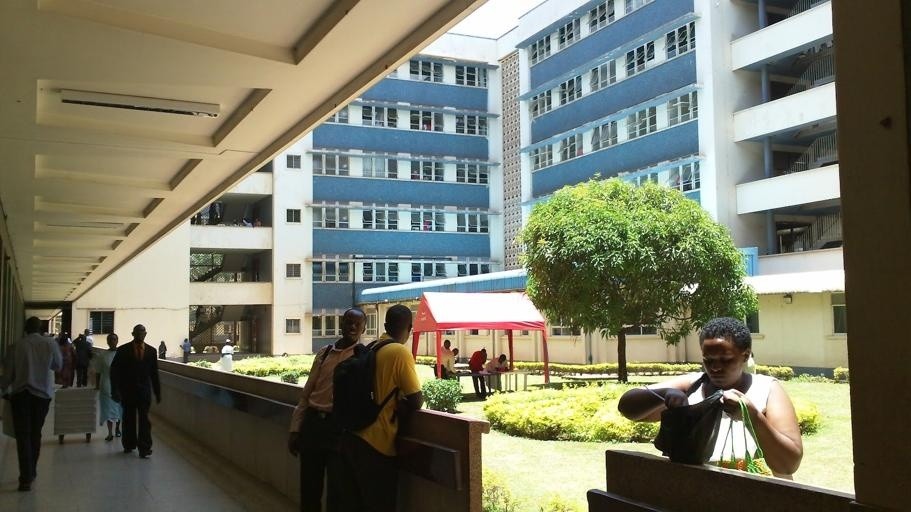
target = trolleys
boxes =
[53,384,100,445]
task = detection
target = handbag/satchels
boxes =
[709,399,776,481]
[653,371,726,466]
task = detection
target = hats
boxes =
[226,339,231,343]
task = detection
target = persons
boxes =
[339,304,424,511]
[484,354,508,393]
[243,217,253,227]
[55,324,162,458]
[159,341,167,360]
[434,340,460,379]
[617,317,804,481]
[468,348,487,397]
[288,308,368,512]
[180,339,191,363]
[452,348,458,356]
[221,339,234,371]
[0,316,64,491]
[411,169,419,179]
[254,217,261,227]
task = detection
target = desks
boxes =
[455,370,533,396]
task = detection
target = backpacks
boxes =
[328,339,409,436]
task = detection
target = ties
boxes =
[135,344,143,361]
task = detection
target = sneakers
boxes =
[105,431,154,458]
[16,470,38,493]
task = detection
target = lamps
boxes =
[61,90,220,119]
[783,295,792,304]
